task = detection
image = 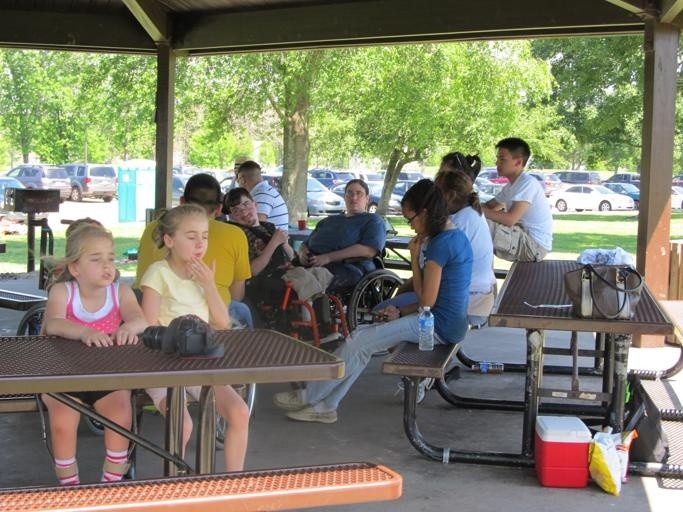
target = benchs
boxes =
[1,462,403,512]
[383,258,508,279]
[381,321,472,448]
[3,384,248,414]
[0,288,47,311]
[663,320,682,373]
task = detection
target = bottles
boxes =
[471,361,504,373]
[418,305,434,350]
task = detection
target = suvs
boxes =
[53,160,118,204]
[5,162,72,206]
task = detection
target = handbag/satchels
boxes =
[563,263,644,321]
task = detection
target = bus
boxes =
[0,174,26,208]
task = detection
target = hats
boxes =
[227,157,250,172]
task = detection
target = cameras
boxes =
[142,315,206,356]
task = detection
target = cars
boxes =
[331,180,404,219]
[305,173,346,218]
[545,183,635,212]
[526,165,682,209]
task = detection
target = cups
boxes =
[296,213,308,229]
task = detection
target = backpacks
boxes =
[600,373,670,463]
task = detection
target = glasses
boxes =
[406,209,423,226]
[344,190,366,198]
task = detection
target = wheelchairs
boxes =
[310,238,403,359]
[85,317,257,451]
[10,299,46,335]
[240,252,348,347]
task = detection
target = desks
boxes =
[3,329,345,473]
[488,260,675,465]
[287,232,413,249]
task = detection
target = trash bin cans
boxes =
[118,159,156,222]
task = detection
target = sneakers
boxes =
[272,389,311,410]
[285,405,337,424]
[393,375,436,405]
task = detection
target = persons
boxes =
[372,171,496,403]
[273,180,474,424]
[290,179,386,296]
[134,173,252,331]
[139,204,250,474]
[38,223,146,487]
[396,152,494,323]
[238,163,290,248]
[478,137,553,264]
[224,186,341,344]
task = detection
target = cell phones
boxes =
[369,310,388,318]
